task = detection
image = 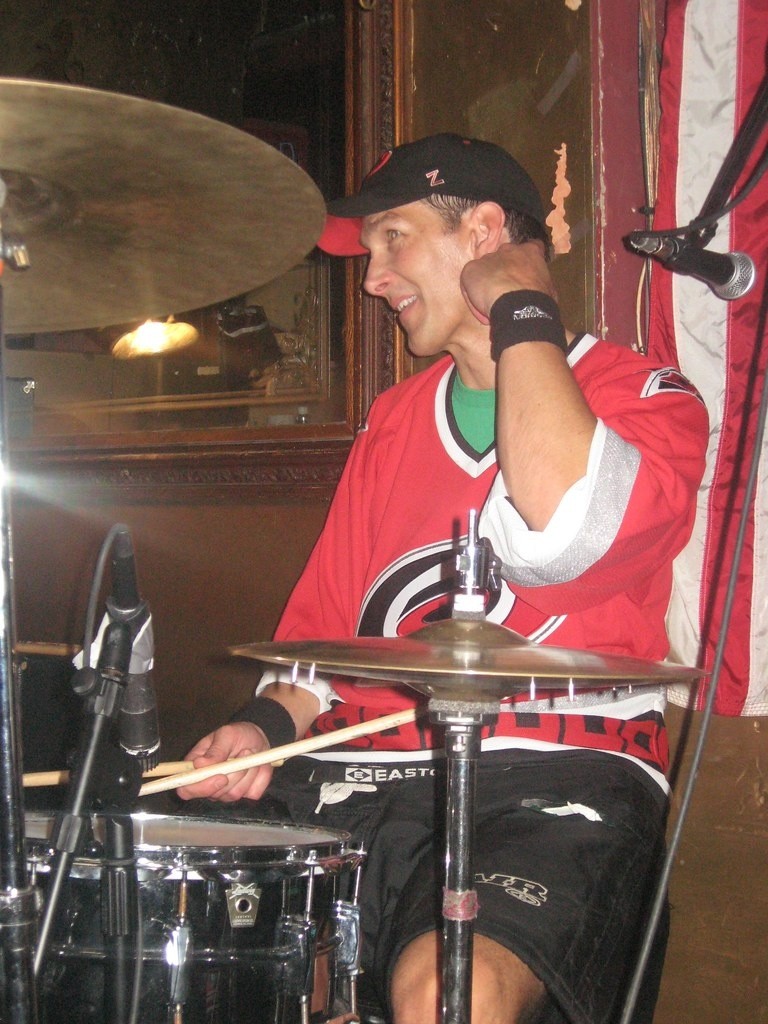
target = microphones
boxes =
[112,531,162,770]
[629,231,756,300]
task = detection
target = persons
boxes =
[176,131,711,1024]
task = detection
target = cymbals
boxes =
[225,616,711,701]
[0,74,328,337]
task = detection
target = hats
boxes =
[316,133,551,256]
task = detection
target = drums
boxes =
[19,809,367,1024]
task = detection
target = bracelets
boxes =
[488,289,569,363]
[229,696,297,748]
